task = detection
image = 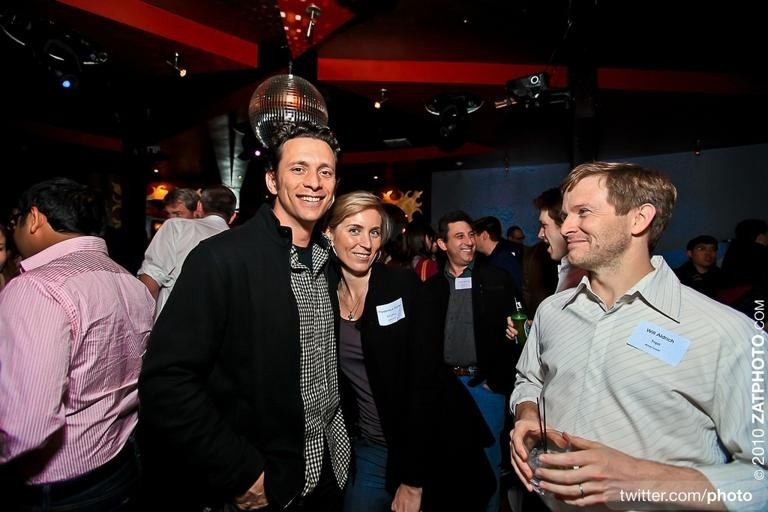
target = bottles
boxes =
[511,295,529,346]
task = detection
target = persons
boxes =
[0,122,767,512]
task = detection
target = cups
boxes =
[521,427,573,497]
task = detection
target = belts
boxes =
[450,366,479,377]
